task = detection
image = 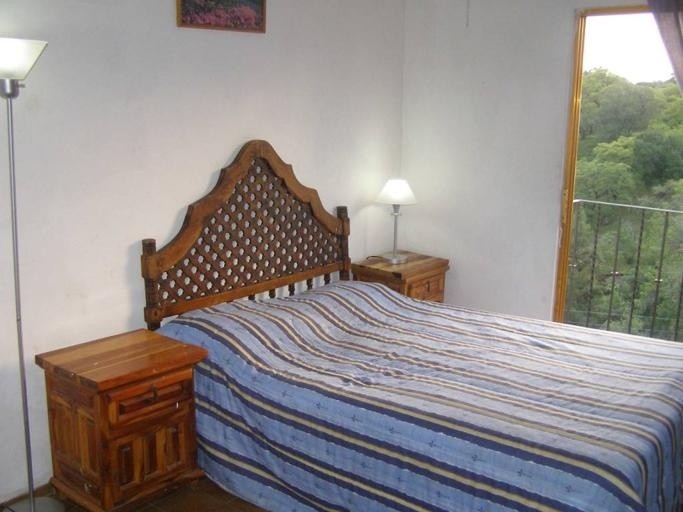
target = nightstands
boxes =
[32,327,208,512]
[351,245,450,306]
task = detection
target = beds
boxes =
[138,138,683,509]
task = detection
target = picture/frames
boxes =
[174,1,267,36]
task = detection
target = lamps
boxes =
[373,176,418,266]
[0,35,65,510]
